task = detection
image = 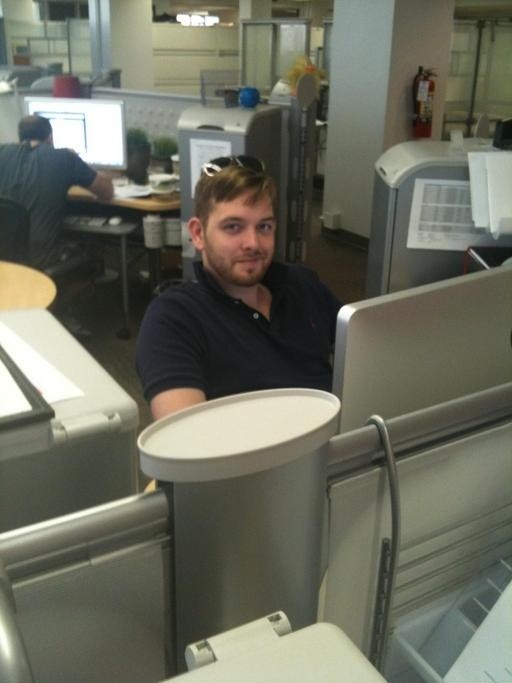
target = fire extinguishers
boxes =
[412,65,438,137]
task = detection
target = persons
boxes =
[134,157,342,421]
[0,114,113,337]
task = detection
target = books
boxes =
[468,150,511,235]
[114,161,182,203]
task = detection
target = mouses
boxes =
[109,215,123,226]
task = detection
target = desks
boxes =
[71,171,180,342]
[0,259,60,311]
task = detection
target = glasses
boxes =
[200,153,266,177]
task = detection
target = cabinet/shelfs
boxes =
[154,43,238,88]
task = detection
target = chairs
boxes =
[1,197,95,294]
[11,66,83,99]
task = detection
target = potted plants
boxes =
[126,126,178,180]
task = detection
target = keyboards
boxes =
[64,213,107,228]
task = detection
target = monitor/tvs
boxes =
[21,94,128,175]
[333,261,512,436]
